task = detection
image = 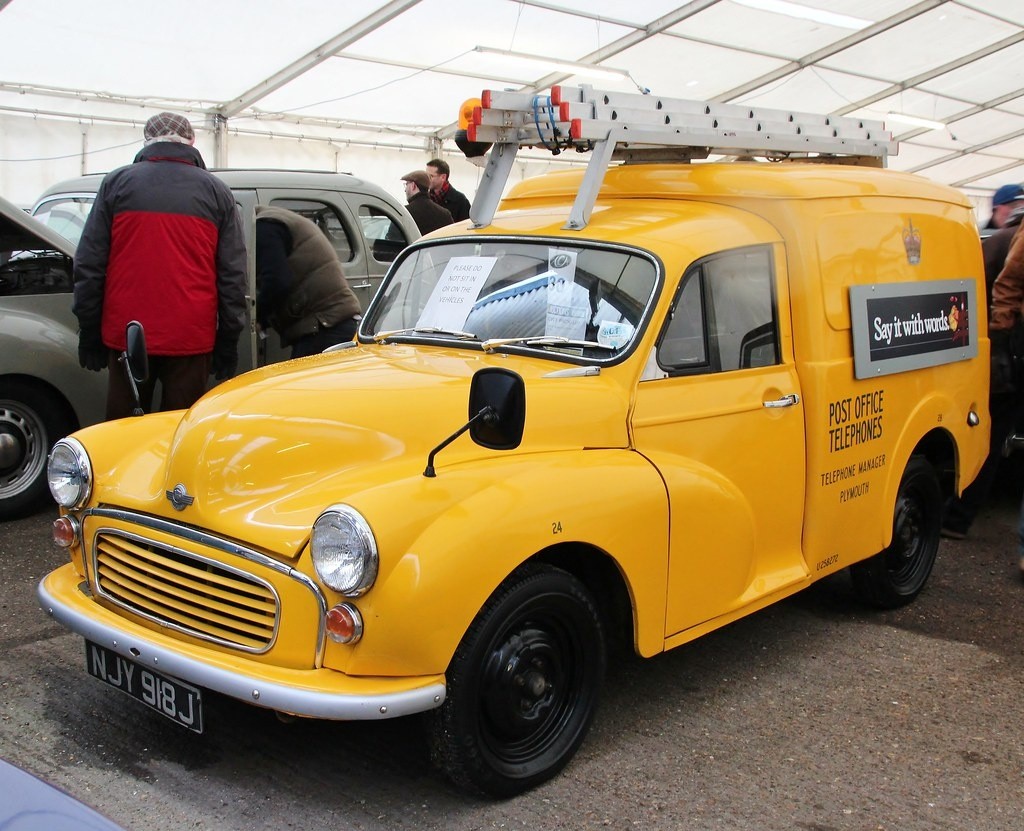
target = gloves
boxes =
[209,336,240,380]
[79,325,109,372]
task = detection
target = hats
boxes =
[401,170,430,189]
[146,112,194,139]
[993,185,1024,206]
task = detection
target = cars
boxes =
[0,199,163,522]
[31,167,439,371]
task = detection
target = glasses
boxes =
[428,173,441,178]
[403,181,413,188]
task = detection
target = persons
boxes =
[385,159,471,243]
[981,184,1024,330]
[255,204,362,360]
[71,112,248,422]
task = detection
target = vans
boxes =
[38,160,994,801]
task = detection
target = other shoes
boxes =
[941,527,966,539]
